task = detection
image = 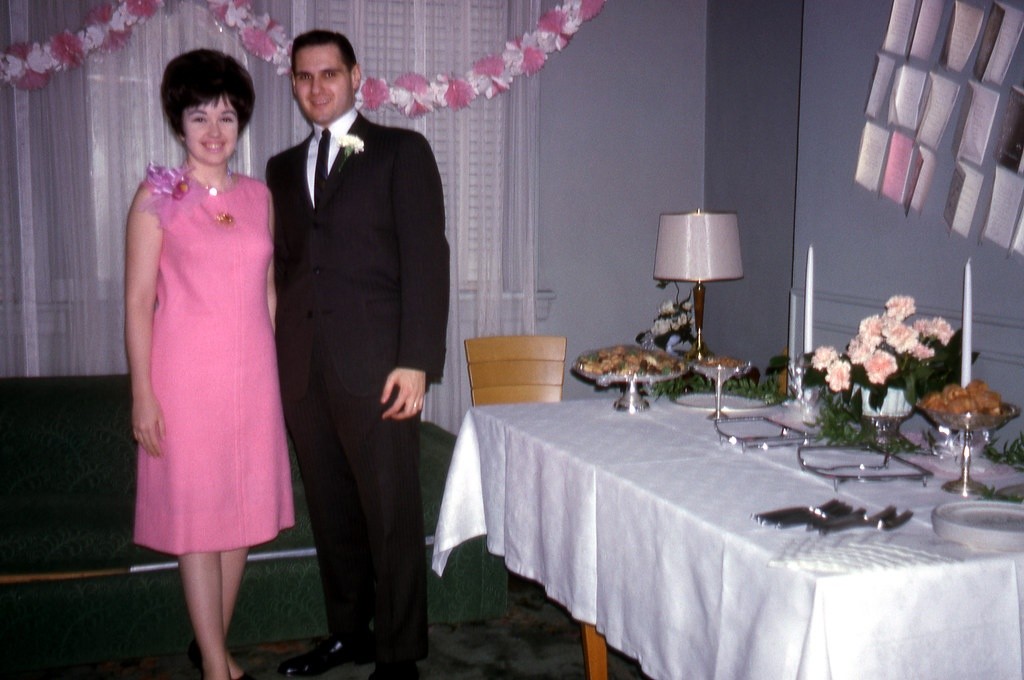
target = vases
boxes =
[853,385,911,450]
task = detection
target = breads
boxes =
[922,378,1003,416]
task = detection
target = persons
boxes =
[266,30,451,680]
[123,47,296,680]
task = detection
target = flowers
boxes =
[804,295,981,412]
[333,133,365,174]
[636,280,697,349]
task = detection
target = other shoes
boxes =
[187,638,257,680]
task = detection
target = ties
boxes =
[314,128,331,213]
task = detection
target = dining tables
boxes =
[431,391,1024,680]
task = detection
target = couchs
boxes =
[1,375,511,660]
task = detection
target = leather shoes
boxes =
[277,629,377,678]
[368,660,420,680]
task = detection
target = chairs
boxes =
[463,337,566,409]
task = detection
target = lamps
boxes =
[654,209,745,380]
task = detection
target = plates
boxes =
[932,499,1024,557]
